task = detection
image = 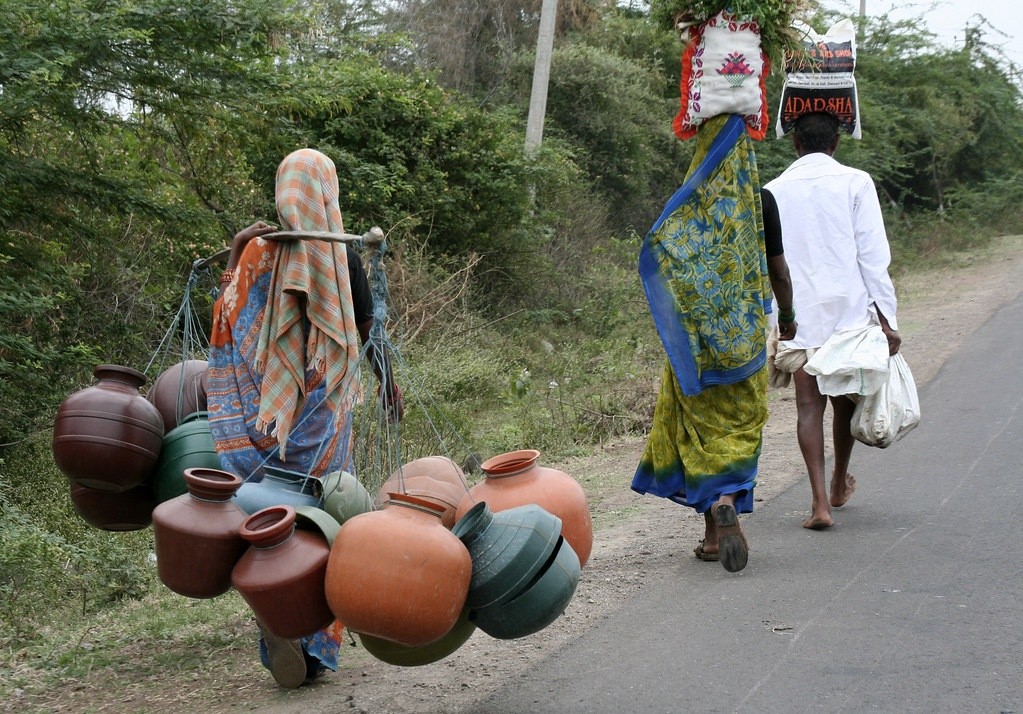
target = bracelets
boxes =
[220,269,236,283]
[777,305,795,323]
[376,384,400,404]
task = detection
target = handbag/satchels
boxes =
[850,348,920,450]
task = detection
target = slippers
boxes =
[693,537,721,561]
[712,501,750,573]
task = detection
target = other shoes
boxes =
[257,618,306,689]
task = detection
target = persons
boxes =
[765,110,902,531]
[630,110,798,572]
[203,149,403,695]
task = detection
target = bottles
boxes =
[52,356,597,667]
